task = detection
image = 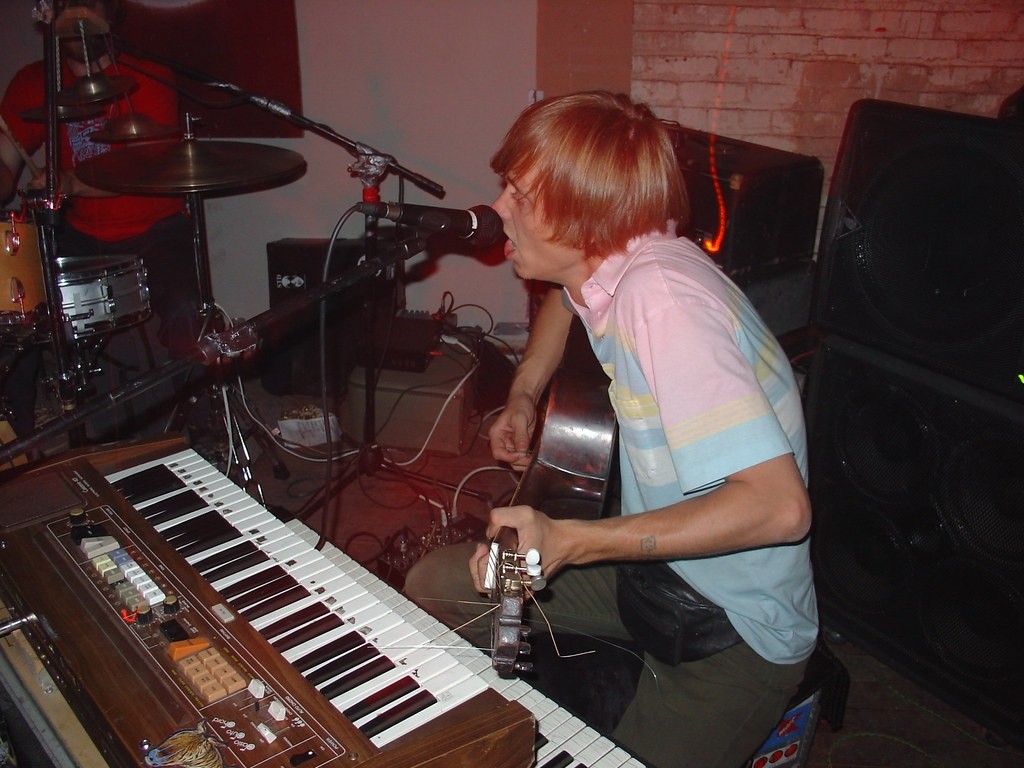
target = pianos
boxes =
[1,431,653,768]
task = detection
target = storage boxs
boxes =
[343,326,484,456]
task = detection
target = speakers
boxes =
[263,238,405,400]
[810,97,1024,407]
[803,337,1024,753]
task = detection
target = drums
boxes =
[33,254,152,344]
[0,208,48,327]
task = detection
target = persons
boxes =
[1,1,239,452]
[402,90,822,768]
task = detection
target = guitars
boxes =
[482,313,620,680]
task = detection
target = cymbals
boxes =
[73,141,305,196]
[55,73,138,106]
[89,113,178,140]
[18,103,103,121]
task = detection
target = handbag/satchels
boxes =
[611,545,745,666]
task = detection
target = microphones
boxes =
[356,202,503,247]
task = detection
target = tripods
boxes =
[161,190,292,479]
[104,38,492,524]
